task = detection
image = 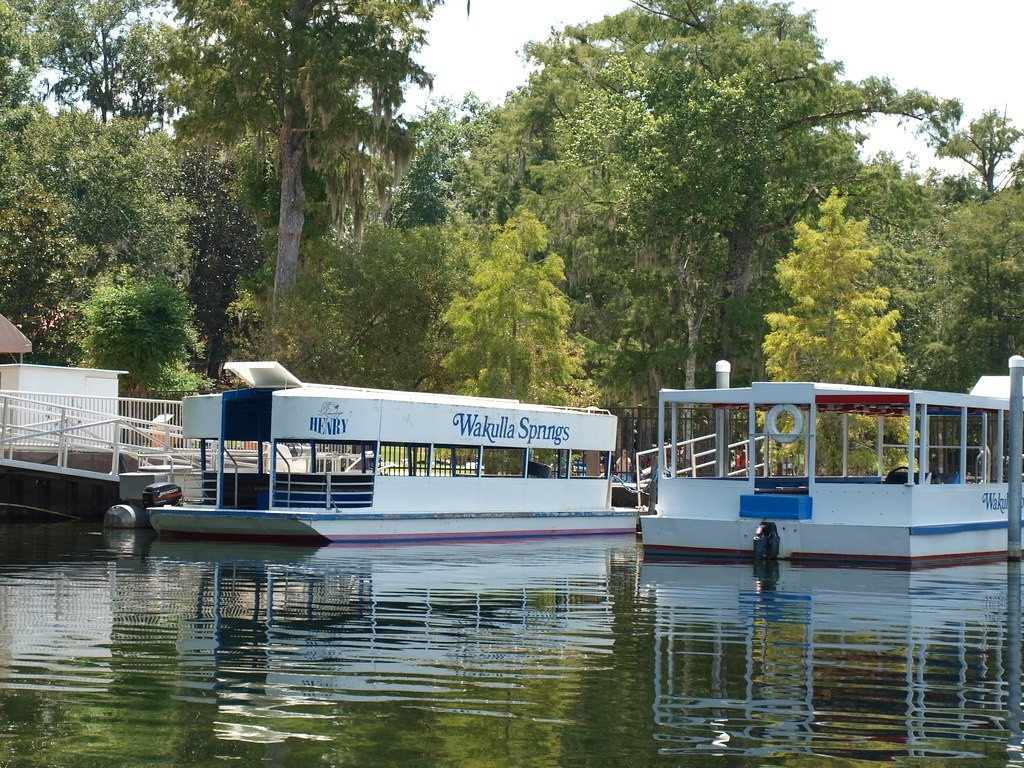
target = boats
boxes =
[637,359,1024,559]
[144,358,644,547]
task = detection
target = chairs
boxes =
[522,461,552,478]
[885,467,917,484]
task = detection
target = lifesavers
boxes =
[764,404,803,443]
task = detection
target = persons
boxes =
[616,449,632,483]
[735,448,747,470]
